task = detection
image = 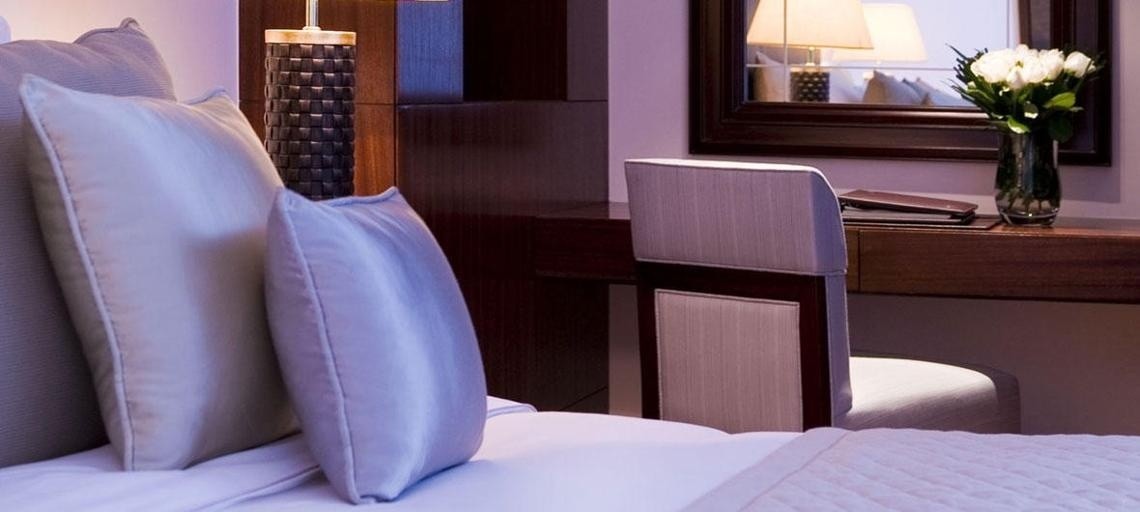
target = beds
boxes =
[2,391,1139,512]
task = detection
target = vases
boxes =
[986,124,1067,228]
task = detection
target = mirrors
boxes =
[687,0,1119,170]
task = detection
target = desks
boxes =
[531,200,1139,304]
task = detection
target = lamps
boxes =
[744,1,873,103]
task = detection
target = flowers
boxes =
[948,40,1107,136]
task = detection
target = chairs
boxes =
[624,157,1025,435]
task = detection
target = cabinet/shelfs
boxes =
[238,0,610,415]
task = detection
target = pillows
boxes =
[752,51,785,102]
[863,70,935,107]
[0,17,177,473]
[19,72,304,473]
[264,186,488,505]
